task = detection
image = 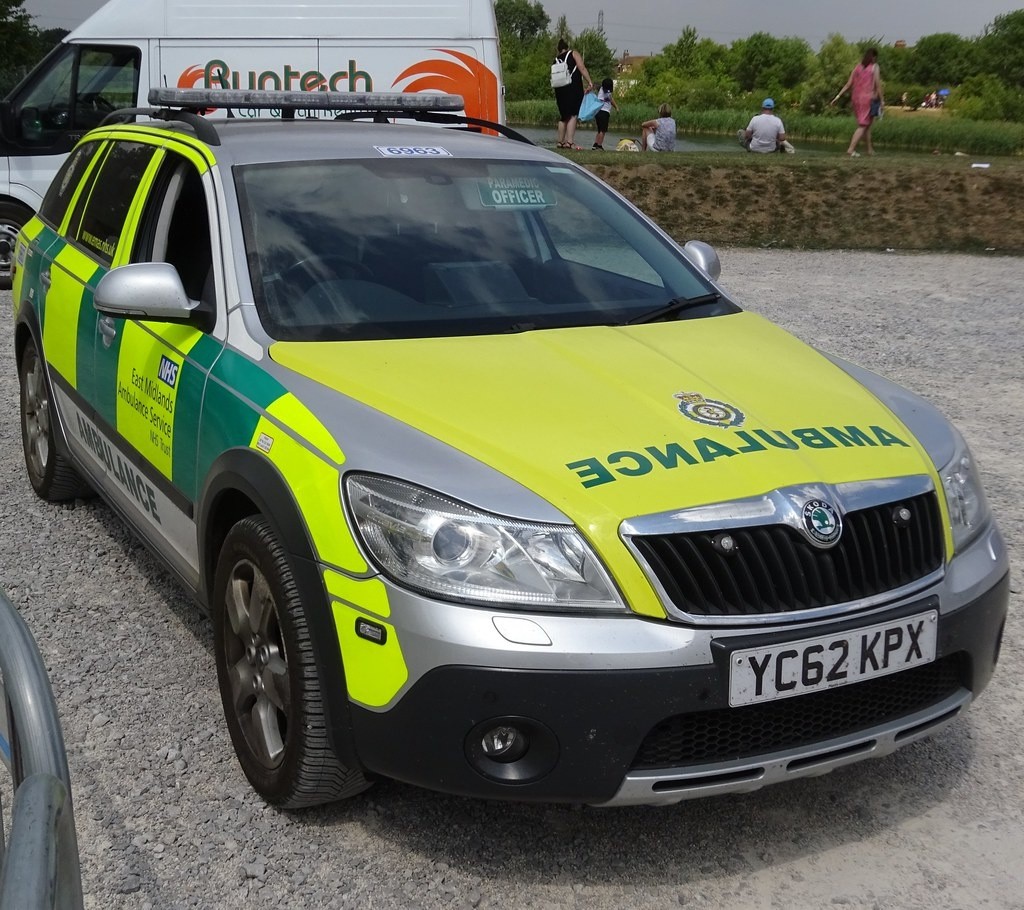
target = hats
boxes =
[762,98,774,108]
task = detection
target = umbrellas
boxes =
[938,89,950,95]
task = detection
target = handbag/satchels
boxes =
[577,91,604,122]
[870,99,884,116]
[615,138,642,152]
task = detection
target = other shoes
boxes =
[591,143,605,151]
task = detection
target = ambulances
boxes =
[15,85,1011,816]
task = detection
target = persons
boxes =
[830,47,884,158]
[642,104,676,152]
[902,89,947,109]
[551,38,593,149]
[738,98,786,153]
[593,77,620,150]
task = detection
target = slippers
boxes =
[848,150,860,156]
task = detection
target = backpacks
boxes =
[551,49,577,87]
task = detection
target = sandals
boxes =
[565,142,578,148]
[556,142,564,147]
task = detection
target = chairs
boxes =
[418,226,541,306]
[200,214,310,325]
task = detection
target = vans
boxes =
[0,0,509,293]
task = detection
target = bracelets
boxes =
[836,95,840,99]
